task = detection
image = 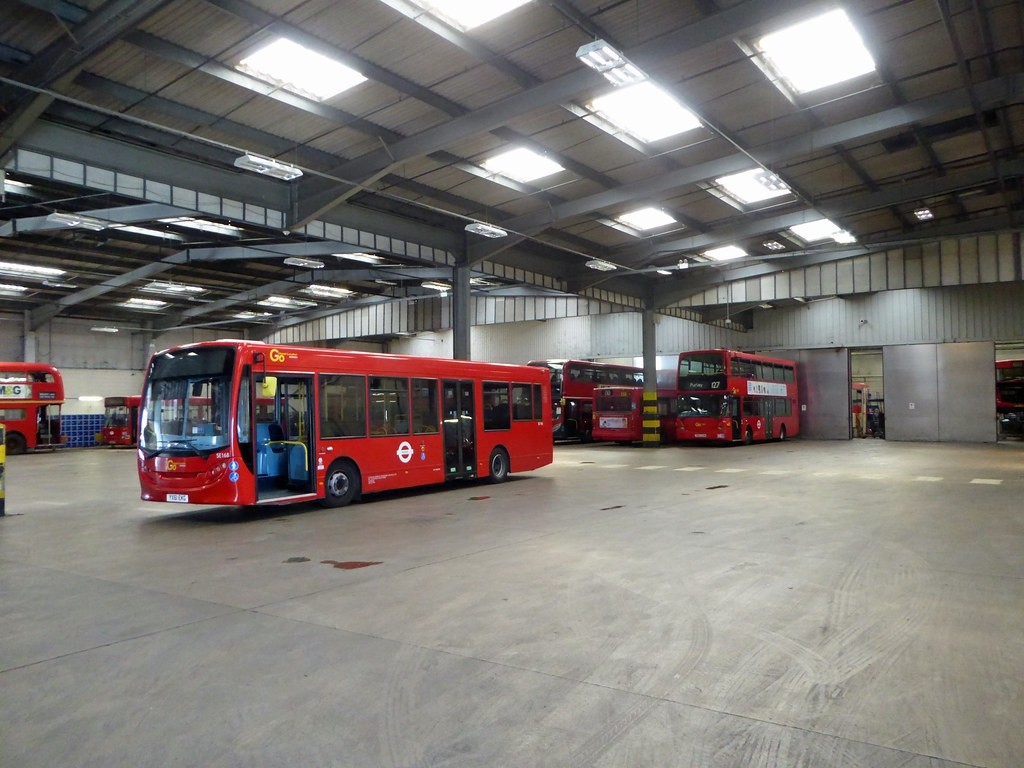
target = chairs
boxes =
[638,378,642,385]
[571,374,576,380]
[634,378,637,385]
[485,403,494,422]
[627,377,632,384]
[613,377,619,385]
[522,397,531,418]
[497,399,509,420]
[514,400,524,420]
[551,375,558,382]
[599,375,605,381]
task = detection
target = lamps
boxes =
[89,326,118,333]
[575,38,648,86]
[45,212,107,232]
[464,222,508,239]
[420,280,451,291]
[233,154,303,181]
[913,206,934,222]
[283,256,325,269]
[753,170,792,192]
[762,240,786,251]
[829,228,859,246]
[585,259,617,271]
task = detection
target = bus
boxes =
[0,362,69,455]
[995,358,1024,441]
[312,387,497,424]
[590,384,677,440]
[521,357,646,443]
[138,337,553,509]
[101,394,289,447]
[676,348,800,445]
